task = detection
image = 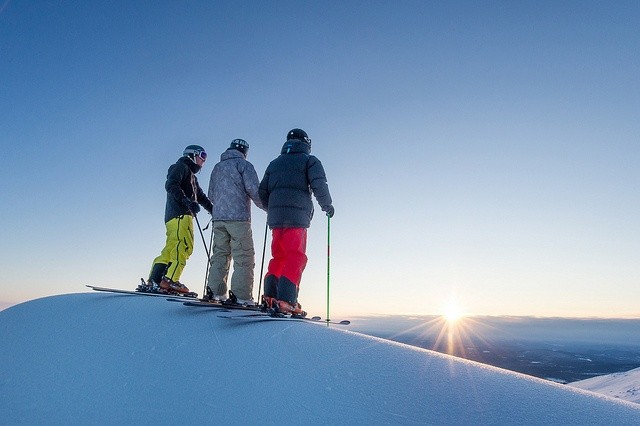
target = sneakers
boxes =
[269,297,306,316]
[262,294,276,313]
[230,295,257,308]
[147,279,159,291]
[207,290,229,303]
[159,277,189,297]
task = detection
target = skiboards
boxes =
[217,315,350,325]
[166,299,307,316]
[85,284,200,299]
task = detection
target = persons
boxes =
[258,128,335,318]
[206,138,269,307]
[149,145,213,292]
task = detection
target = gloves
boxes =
[187,200,200,213]
[323,204,334,218]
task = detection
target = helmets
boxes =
[182,144,206,165]
[229,139,249,157]
[287,128,311,145]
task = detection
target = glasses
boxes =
[199,151,207,162]
